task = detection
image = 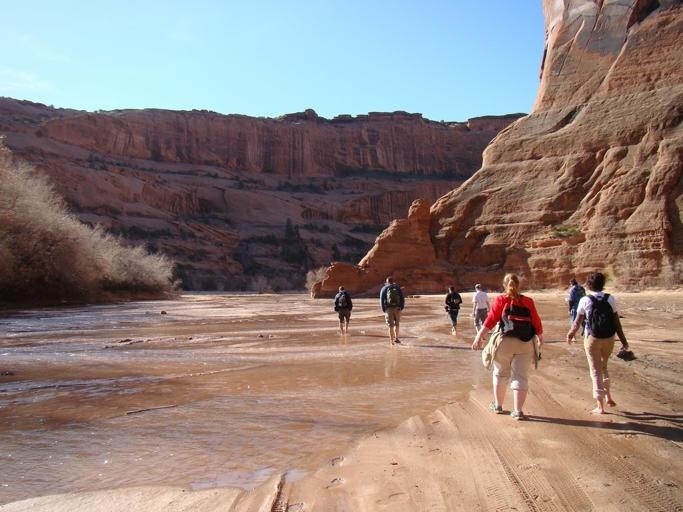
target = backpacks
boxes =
[499,293,536,341]
[383,283,402,306]
[336,292,348,308]
[584,291,616,339]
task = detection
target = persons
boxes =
[565,278,586,343]
[471,283,490,333]
[471,273,544,421]
[332,285,352,335]
[379,276,404,345]
[444,286,462,333]
[566,272,630,415]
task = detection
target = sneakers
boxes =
[616,349,636,362]
[510,409,524,420]
[489,401,503,414]
